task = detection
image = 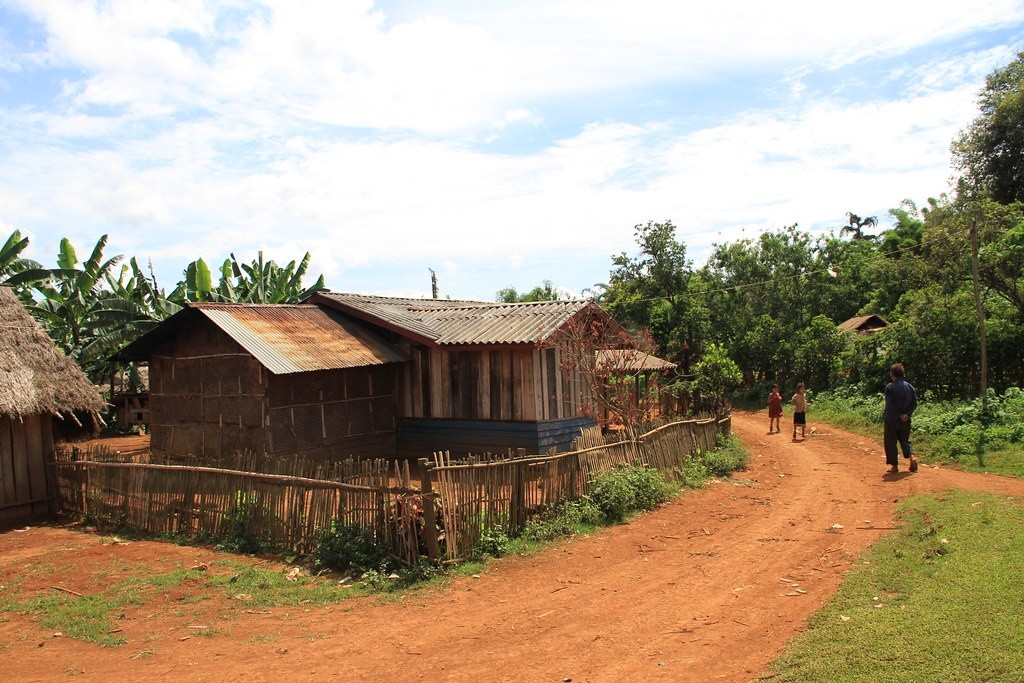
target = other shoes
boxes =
[801,434,805,437]
[770,429,775,434]
[887,468,900,473]
[793,434,796,438]
[909,458,918,471]
[776,426,781,432]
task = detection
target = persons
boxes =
[792,383,811,438]
[884,363,918,472]
[768,384,783,432]
